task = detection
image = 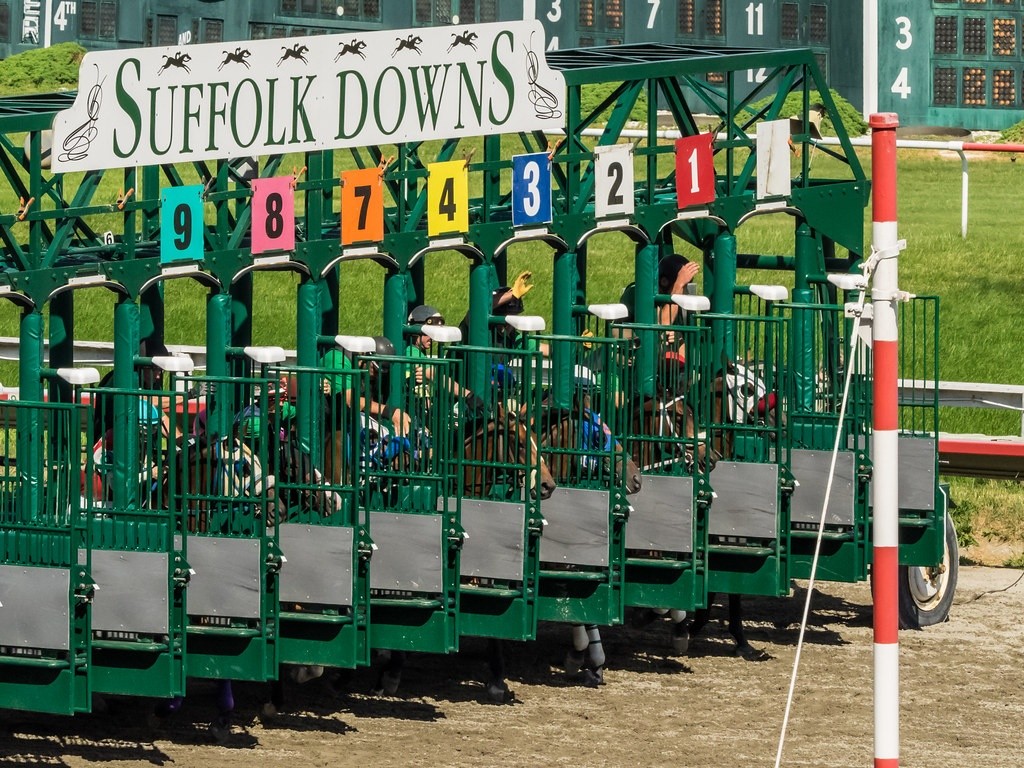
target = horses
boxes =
[686,353,788,660]
[324,406,422,700]
[139,429,288,745]
[447,400,557,704]
[615,393,719,658]
[532,393,643,687]
[258,438,340,726]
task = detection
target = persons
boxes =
[611,254,700,374]
[64,341,217,515]
[442,270,534,400]
[319,305,482,438]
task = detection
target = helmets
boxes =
[407,305,445,325]
[659,254,689,277]
[493,287,524,312]
[369,337,395,372]
[139,400,159,424]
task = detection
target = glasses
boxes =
[616,338,641,351]
